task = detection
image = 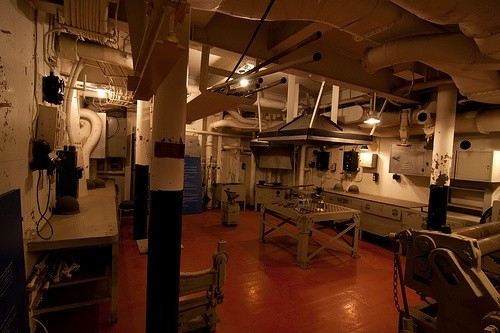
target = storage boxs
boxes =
[358,152,377,169]
[315,152,330,173]
[342,151,358,173]
[36,104,62,149]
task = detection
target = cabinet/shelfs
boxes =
[214,182,247,210]
[388,143,500,183]
[252,186,288,211]
[321,188,482,245]
[104,117,129,160]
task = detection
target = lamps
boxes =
[363,92,381,124]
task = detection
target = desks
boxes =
[259,200,361,270]
